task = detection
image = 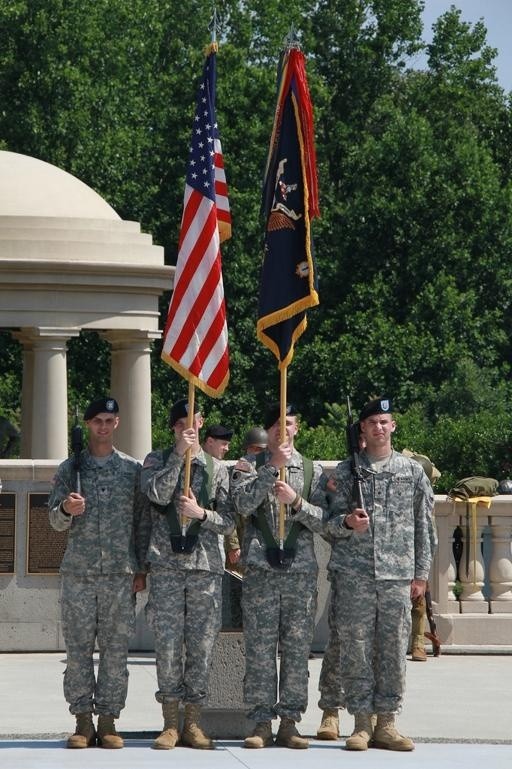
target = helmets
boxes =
[242,427,269,449]
[412,452,433,480]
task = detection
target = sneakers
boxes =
[410,645,427,660]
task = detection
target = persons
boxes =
[401,448,440,663]
[223,426,268,628]
[140,395,237,751]
[46,392,150,750]
[318,396,439,754]
[203,423,233,460]
[228,398,328,750]
[317,418,379,742]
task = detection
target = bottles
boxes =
[499,477,512,495]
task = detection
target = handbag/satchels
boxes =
[450,475,499,499]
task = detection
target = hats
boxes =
[83,397,119,420]
[170,398,199,427]
[263,402,299,429]
[360,399,393,421]
[205,424,233,440]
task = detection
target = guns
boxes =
[346,395,367,517]
[72,403,83,493]
[425,580,440,655]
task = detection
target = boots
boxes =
[346,712,376,748]
[373,710,415,749]
[277,719,310,748]
[68,713,95,747]
[244,719,274,747]
[153,704,181,748]
[318,706,340,740]
[181,703,213,748]
[98,715,124,748]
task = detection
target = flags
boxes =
[161,41,234,401]
[254,41,322,373]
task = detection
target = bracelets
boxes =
[289,493,301,509]
[59,499,72,517]
[197,510,207,521]
[344,517,353,531]
